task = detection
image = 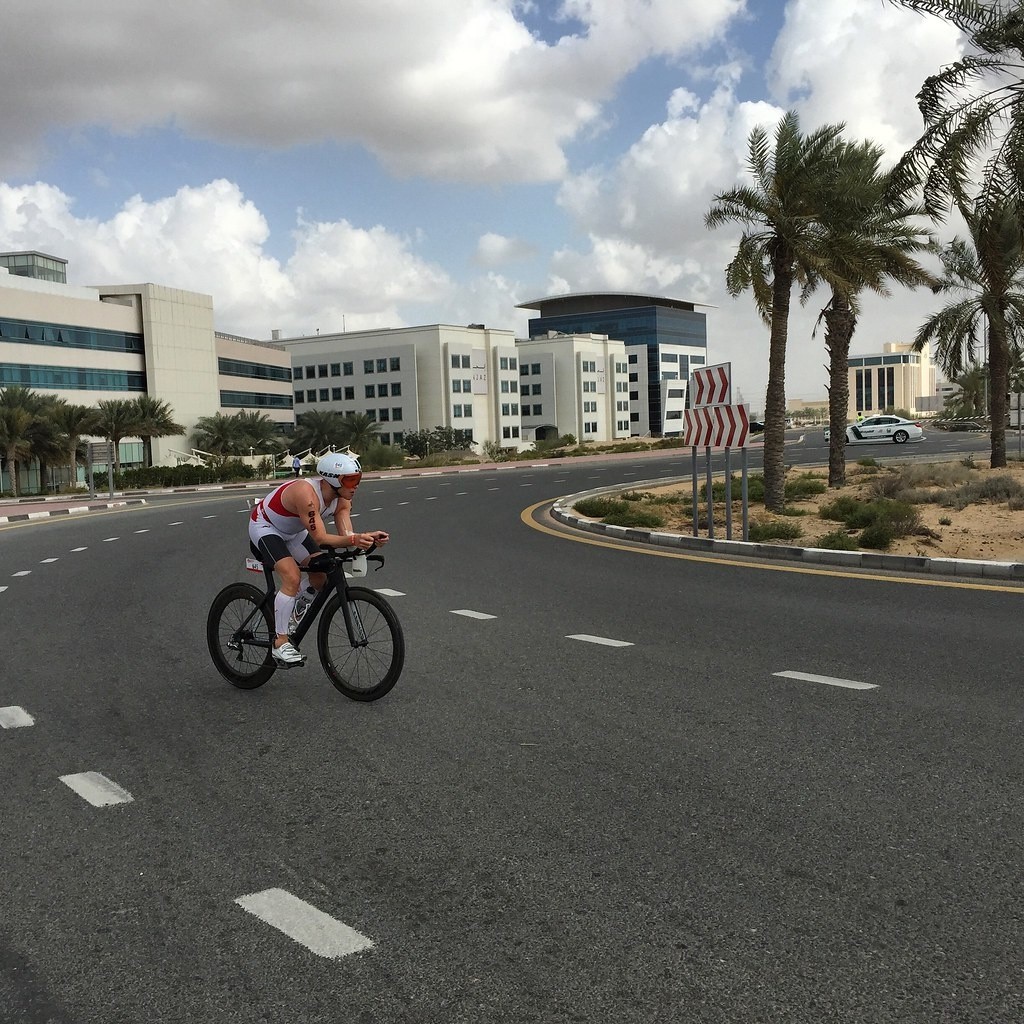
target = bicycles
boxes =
[207,535,406,703]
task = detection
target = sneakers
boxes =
[289,616,298,634]
[271,641,307,662]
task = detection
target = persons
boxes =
[249,453,390,665]
[856,413,864,421]
[292,456,301,477]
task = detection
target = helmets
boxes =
[317,454,360,487]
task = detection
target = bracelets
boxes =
[352,535,355,545]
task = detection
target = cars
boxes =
[750,422,765,432]
[824,415,923,445]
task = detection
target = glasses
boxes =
[340,473,362,488]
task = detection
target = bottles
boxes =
[352,549,368,577]
[292,586,316,620]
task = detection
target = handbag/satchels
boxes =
[299,468,302,475]
